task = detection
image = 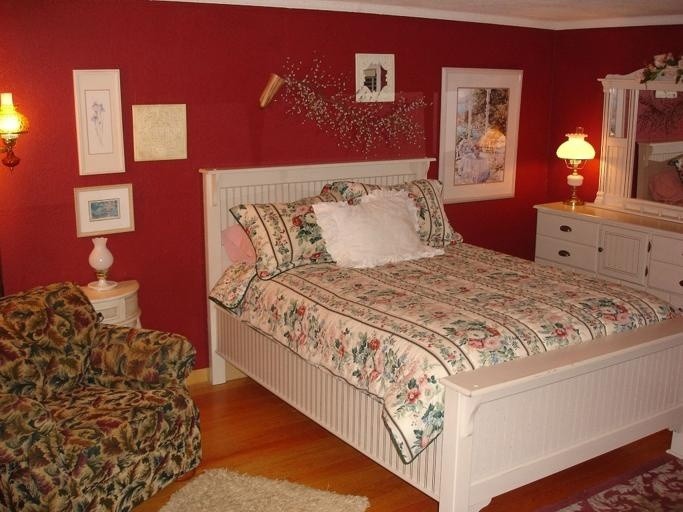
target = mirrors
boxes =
[599,56,682,226]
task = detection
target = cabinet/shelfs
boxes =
[522,198,682,310]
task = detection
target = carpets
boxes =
[554,459,682,512]
[153,465,373,511]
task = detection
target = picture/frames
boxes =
[71,180,138,239]
[437,66,525,204]
[353,51,396,104]
[69,66,130,178]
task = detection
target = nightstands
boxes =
[77,278,145,330]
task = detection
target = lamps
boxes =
[0,91,30,171]
[554,125,596,205]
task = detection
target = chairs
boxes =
[0,281,204,510]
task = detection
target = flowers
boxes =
[639,48,682,85]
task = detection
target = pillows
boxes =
[312,186,442,270]
[647,167,682,207]
[333,175,462,252]
[224,183,338,280]
[219,225,256,266]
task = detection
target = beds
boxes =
[634,139,683,206]
[196,159,682,510]
